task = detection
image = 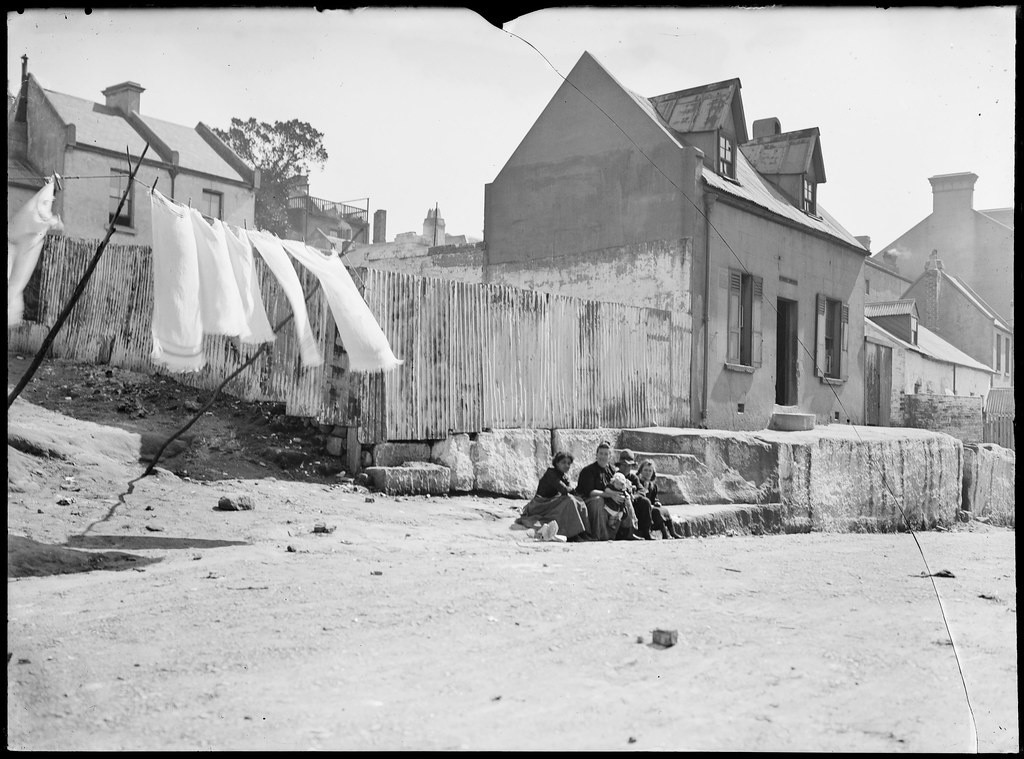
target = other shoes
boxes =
[567,534,596,542]
[643,535,655,540]
[624,534,644,540]
[607,524,616,530]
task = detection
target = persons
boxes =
[522,441,684,542]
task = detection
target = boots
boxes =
[660,525,684,539]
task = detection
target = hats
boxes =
[610,473,627,491]
[614,449,638,469]
